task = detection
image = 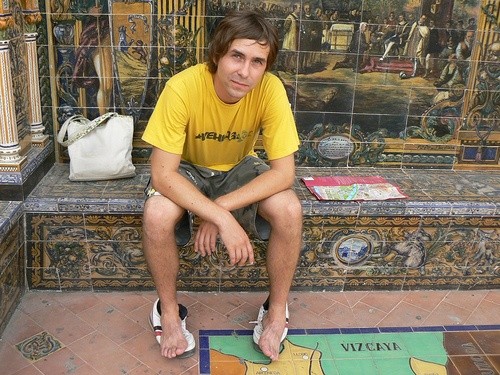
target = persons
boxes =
[141,10,301,362]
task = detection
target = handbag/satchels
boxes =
[56,112,136,181]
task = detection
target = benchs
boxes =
[0,200,25,338]
[25,164,500,292]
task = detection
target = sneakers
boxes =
[249,294,289,352]
[148,298,196,359]
